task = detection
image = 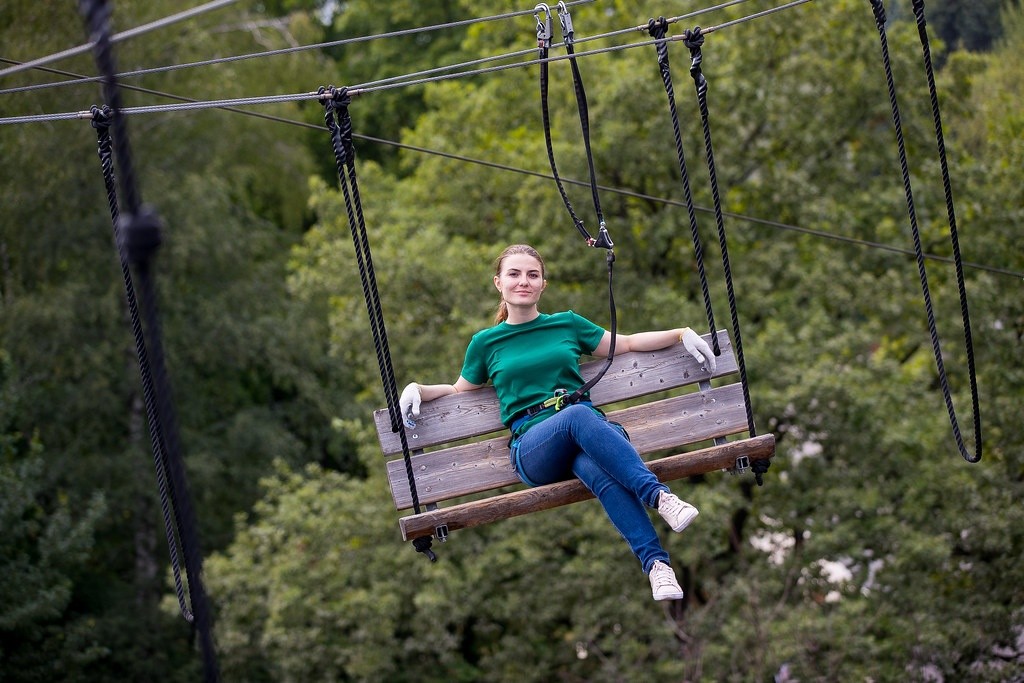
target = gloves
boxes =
[679,327,716,374]
[399,382,422,430]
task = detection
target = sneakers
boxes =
[658,490,698,533]
[648,559,683,601]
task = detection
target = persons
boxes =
[399,243,716,600]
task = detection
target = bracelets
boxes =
[679,326,690,342]
